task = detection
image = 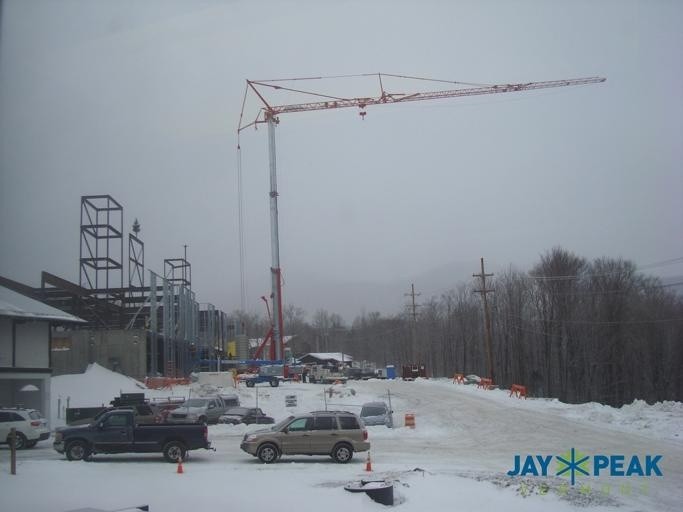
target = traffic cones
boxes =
[176,455,185,474]
[364,450,372,472]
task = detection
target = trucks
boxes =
[308,366,349,385]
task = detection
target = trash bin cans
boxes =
[386,365,394,379]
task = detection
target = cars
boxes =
[345,367,378,381]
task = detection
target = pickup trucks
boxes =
[167,393,239,425]
[67,405,199,427]
[217,407,274,425]
[51,406,217,464]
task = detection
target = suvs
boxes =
[239,409,371,467]
[359,400,393,428]
[0,403,52,451]
